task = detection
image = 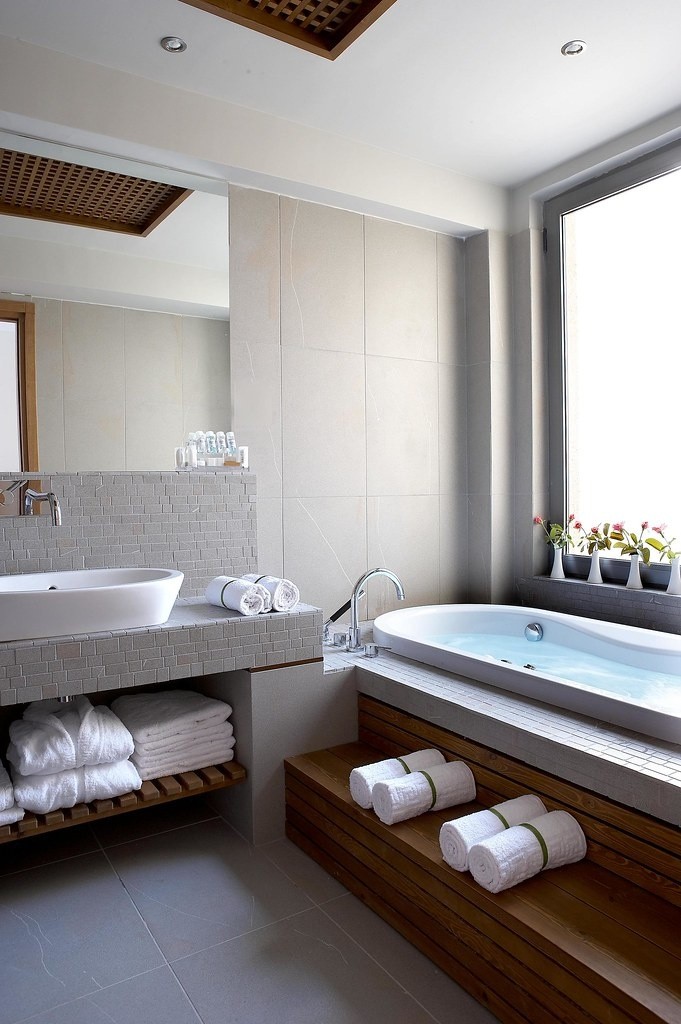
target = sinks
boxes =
[0,568,185,642]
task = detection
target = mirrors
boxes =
[1,150,230,471]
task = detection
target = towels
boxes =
[205,574,272,616]
[113,689,236,781]
[371,761,477,826]
[240,574,300,612]
[349,748,447,809]
[467,809,588,895]
[438,793,549,872]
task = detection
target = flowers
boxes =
[534,514,574,549]
[645,523,681,564]
[573,521,613,554]
[610,520,651,565]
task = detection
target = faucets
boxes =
[348,568,406,650]
[26,487,62,528]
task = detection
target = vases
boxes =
[551,548,565,579]
[625,554,643,589]
[666,559,681,594]
[587,550,603,583]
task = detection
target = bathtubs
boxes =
[368,599,681,754]
